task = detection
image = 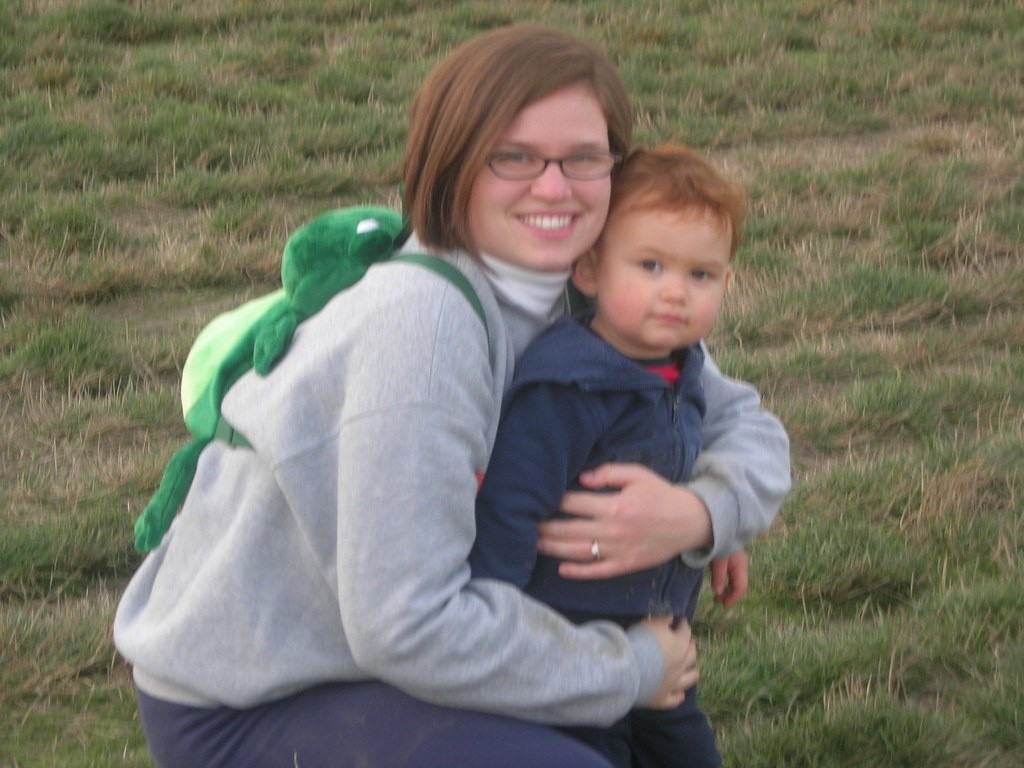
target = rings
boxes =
[590,537,601,562]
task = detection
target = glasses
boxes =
[484,151,621,180]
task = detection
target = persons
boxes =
[114,19,790,767]
[470,143,752,768]
